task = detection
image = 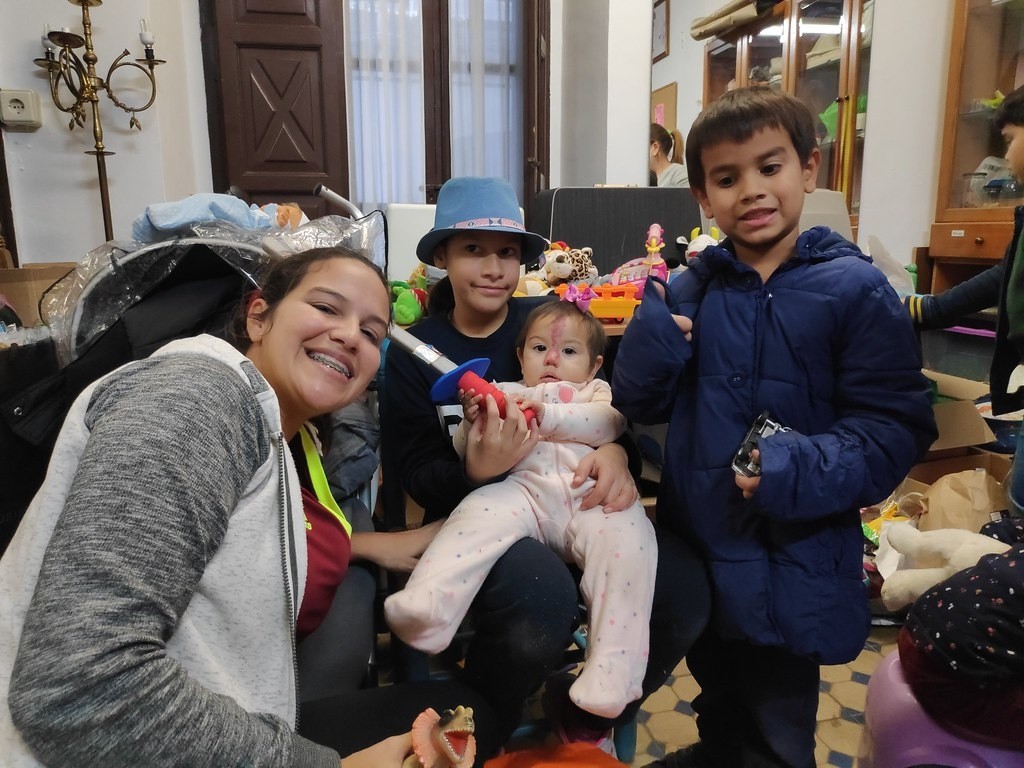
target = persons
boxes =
[0,249,448,768]
[383,285,658,718]
[648,123,690,188]
[378,176,644,768]
[899,85,1024,416]
[612,85,940,768]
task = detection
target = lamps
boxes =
[31,0,167,243]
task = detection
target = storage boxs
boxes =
[920,368,999,462]
[0,262,79,329]
[898,451,1014,498]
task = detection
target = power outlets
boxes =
[0,89,42,128]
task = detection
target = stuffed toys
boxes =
[388,262,429,326]
[881,523,1013,612]
[512,240,598,296]
[666,226,727,285]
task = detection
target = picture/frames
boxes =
[652,0,670,64]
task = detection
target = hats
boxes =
[415,176,546,267]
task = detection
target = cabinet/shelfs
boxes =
[703,0,875,247]
[930,0,1024,302]
